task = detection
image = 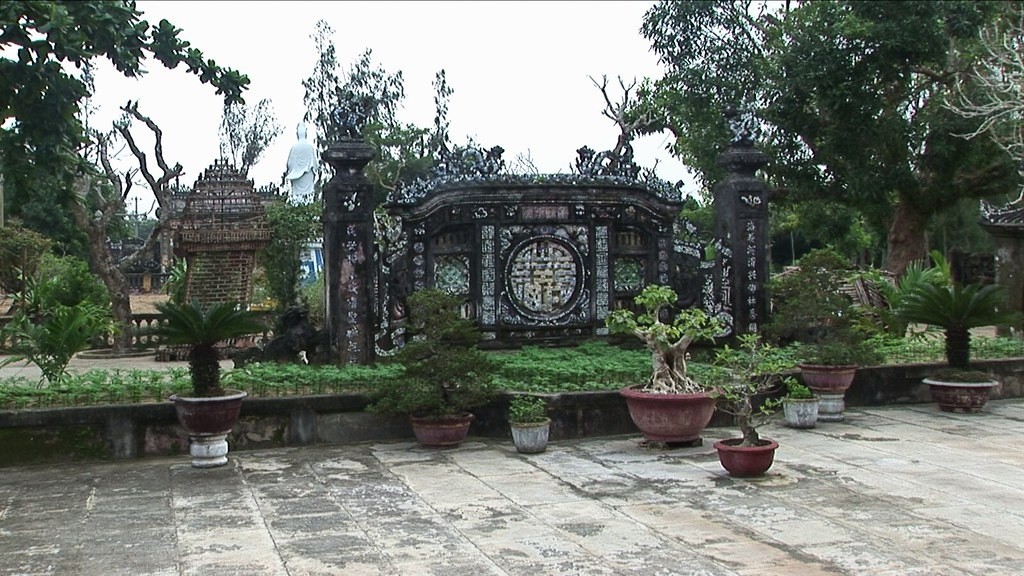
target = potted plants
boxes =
[363,289,508,446]
[604,285,723,445]
[702,329,802,479]
[149,299,272,470]
[888,254,1008,415]
[771,251,870,393]
[781,378,820,430]
[510,394,553,454]
